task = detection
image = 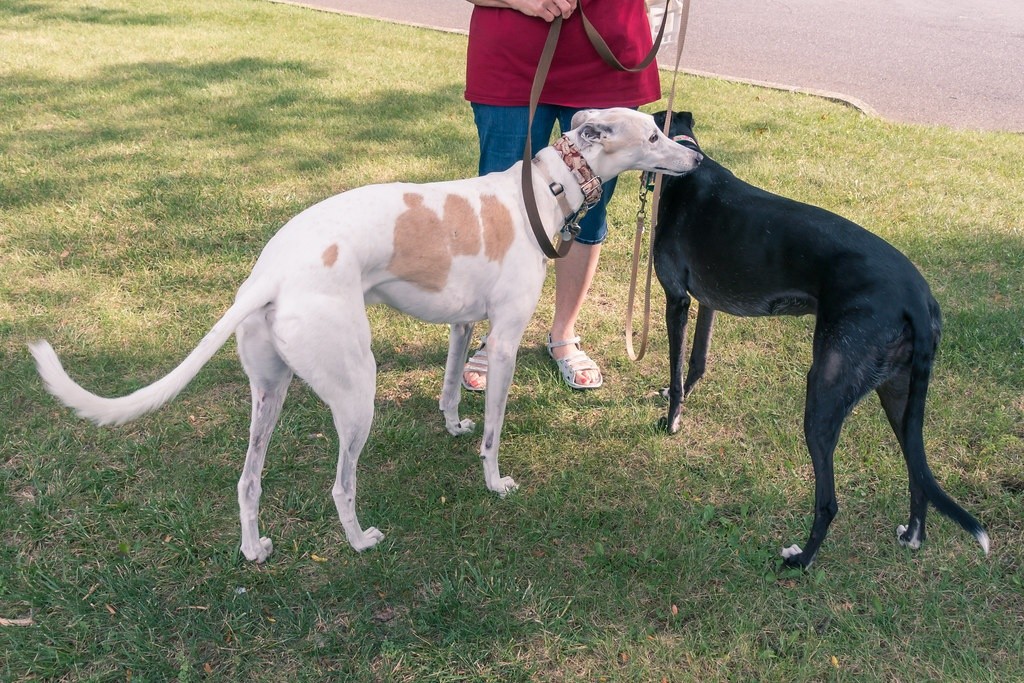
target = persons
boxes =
[462,0,661,392]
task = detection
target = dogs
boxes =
[638,110,992,572]
[31,105,705,564]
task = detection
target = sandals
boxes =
[547,332,603,390]
[461,335,487,391]
